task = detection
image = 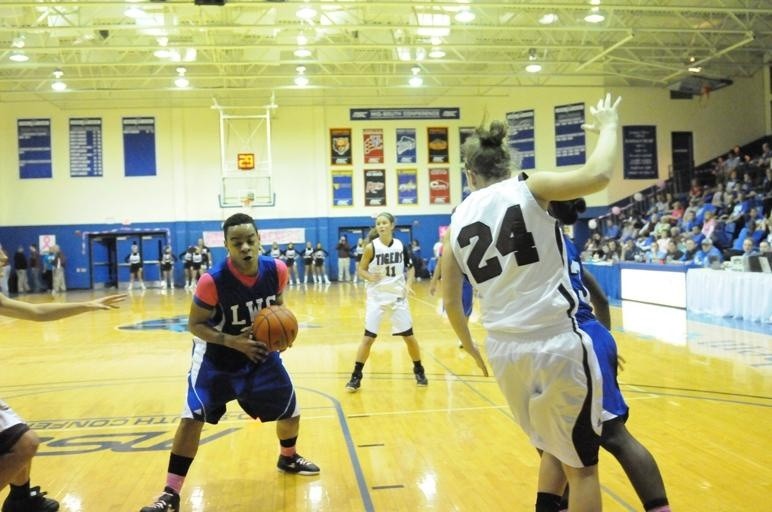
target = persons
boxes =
[259,241,331,286]
[345,212,428,392]
[547,197,670,511]
[430,245,473,349]
[440,93,623,511]
[140,213,320,512]
[160,238,216,289]
[0,400,59,511]
[125,244,146,291]
[337,234,368,282]
[0,243,67,298]
[402,238,428,281]
[575,142,772,272]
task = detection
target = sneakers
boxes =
[345,373,362,391]
[276,454,320,475]
[139,486,179,512]
[1,486,59,512]
[414,366,427,386]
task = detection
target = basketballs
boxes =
[252,306,297,352]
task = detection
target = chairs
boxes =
[600,182,765,267]
